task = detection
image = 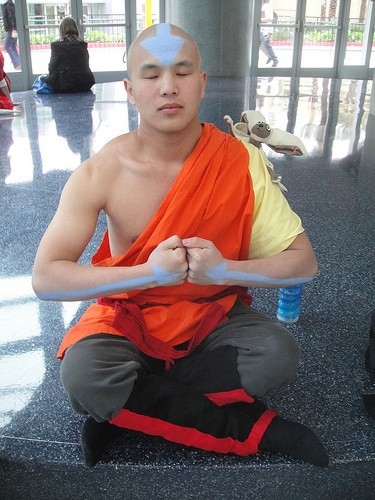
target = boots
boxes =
[108,368,330,467]
[82,344,241,466]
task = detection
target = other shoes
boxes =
[266,57,272,64]
[272,60,279,67]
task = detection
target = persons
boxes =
[1,0,21,70]
[31,23,330,468]
[0,51,23,110]
[259,0,279,67]
[40,18,95,93]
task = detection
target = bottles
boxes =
[275,284,302,322]
